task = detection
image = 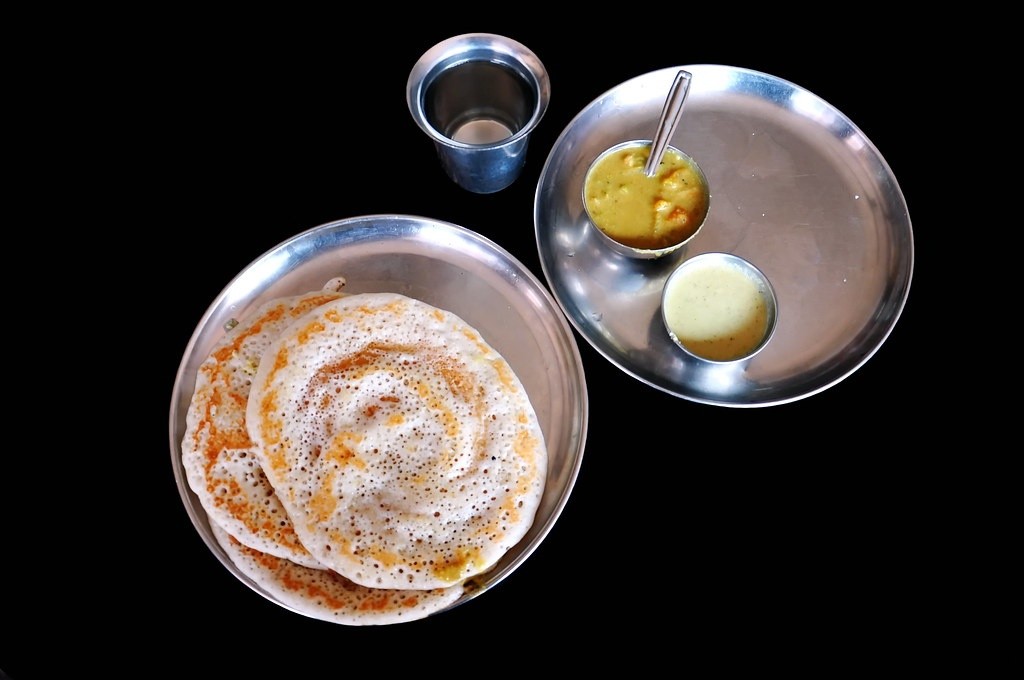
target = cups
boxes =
[406,33,550,195]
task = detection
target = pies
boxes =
[181,292,549,626]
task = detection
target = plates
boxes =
[534,66,913,409]
[169,214,589,623]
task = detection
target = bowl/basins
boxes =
[660,252,778,364]
[582,140,710,261]
